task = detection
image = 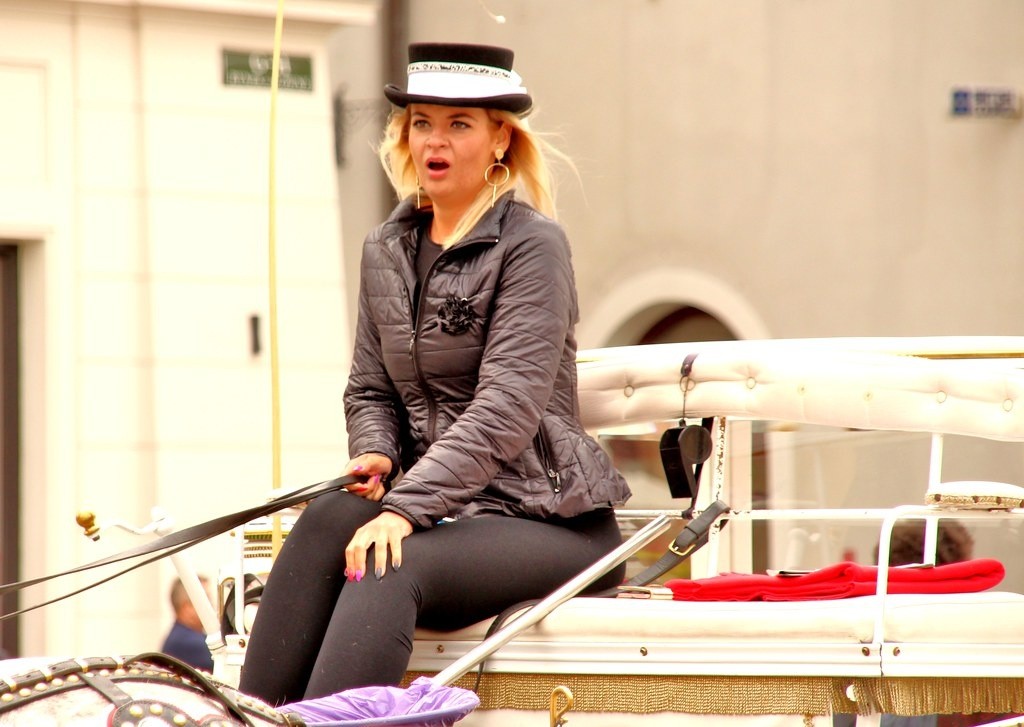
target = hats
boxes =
[383,43,534,120]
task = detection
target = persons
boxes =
[162,576,216,675]
[238,41,631,707]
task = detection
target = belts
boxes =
[472,500,731,694]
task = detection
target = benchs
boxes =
[150,335,1024,727]
